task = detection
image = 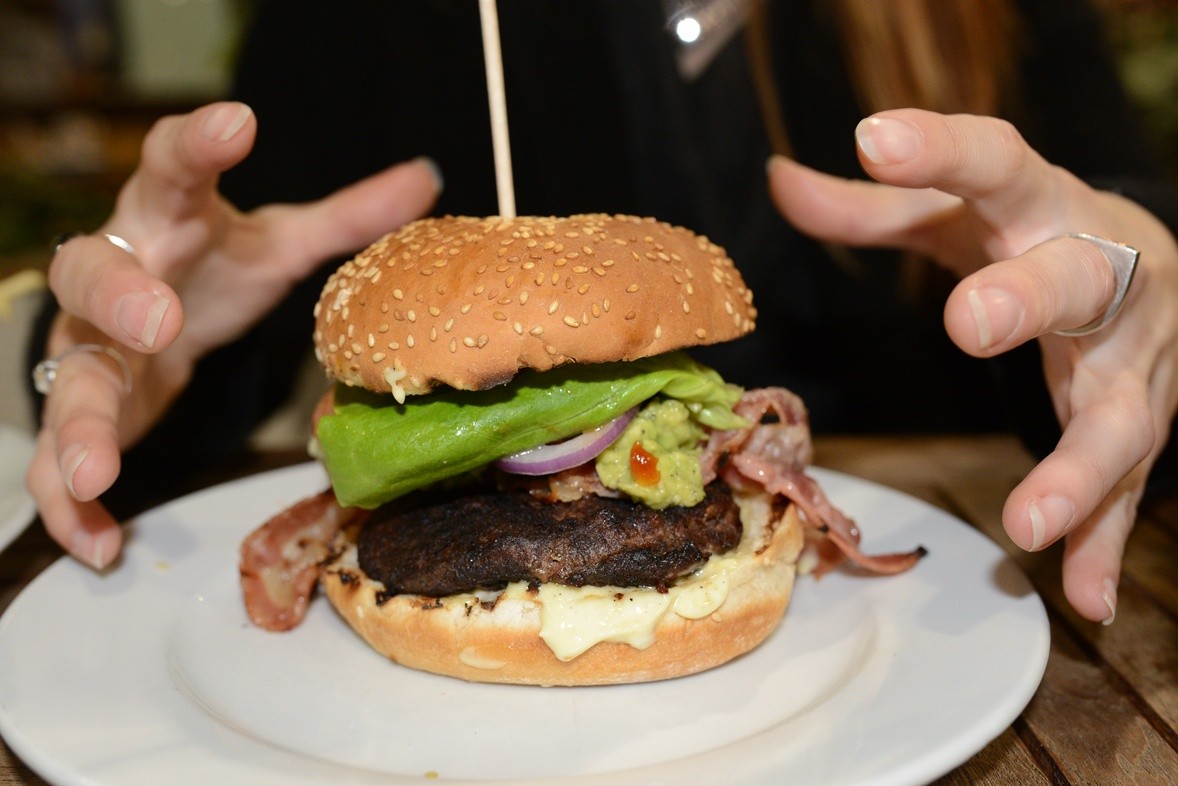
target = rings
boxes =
[1050,231,1141,337]
[33,342,132,396]
[55,233,138,257]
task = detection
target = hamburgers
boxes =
[241,212,930,686]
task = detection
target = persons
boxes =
[23,0,1178,626]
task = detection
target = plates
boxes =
[2,457,1053,786]
